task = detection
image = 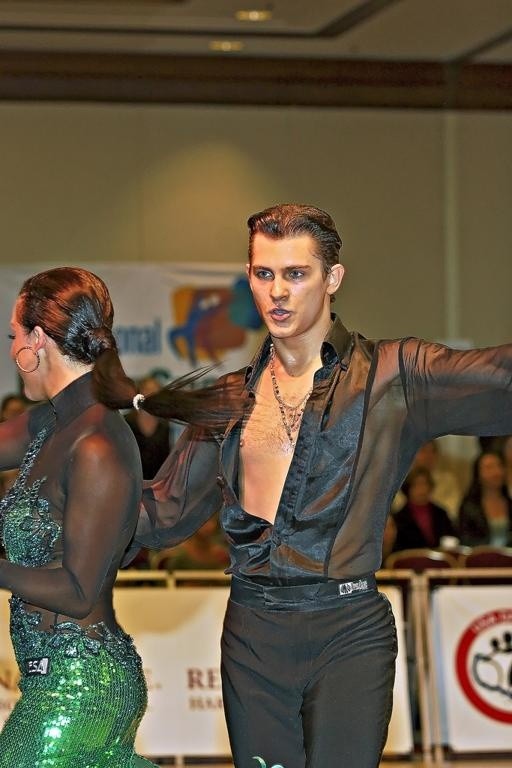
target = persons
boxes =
[122,376,231,571]
[381,433,510,569]
[118,199,512,767]
[1,265,255,766]
[2,395,33,470]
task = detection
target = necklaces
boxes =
[268,342,315,447]
